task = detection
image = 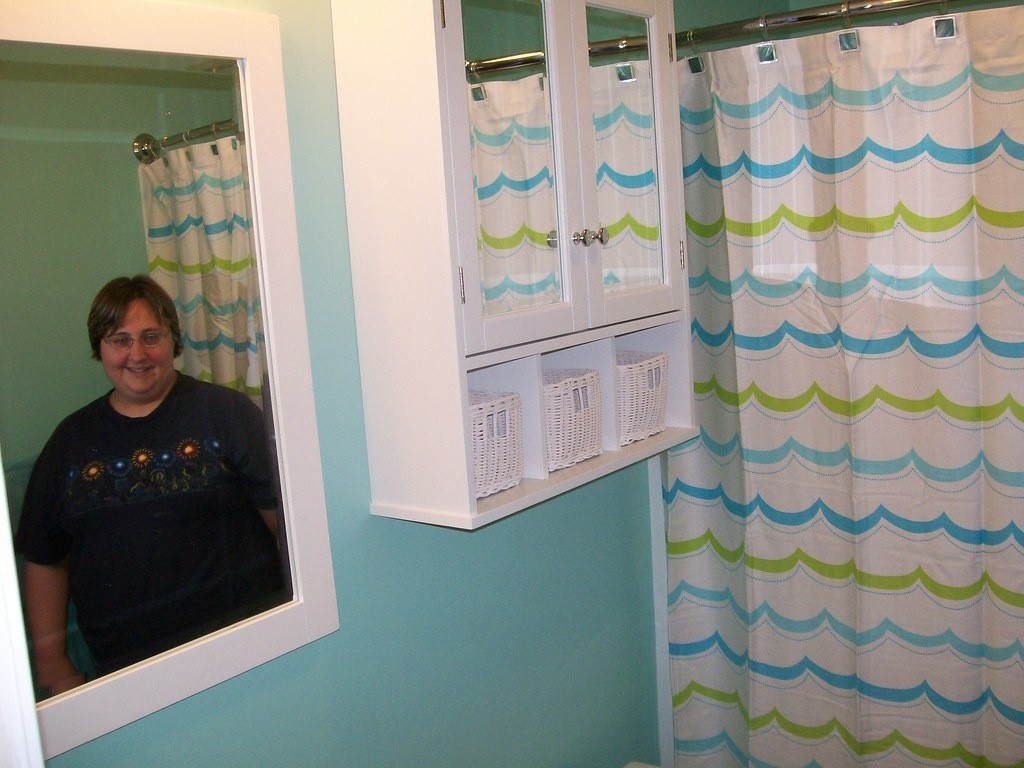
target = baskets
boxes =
[467,390,524,500]
[542,368,603,472]
[616,349,670,446]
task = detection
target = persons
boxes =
[14,274,282,697]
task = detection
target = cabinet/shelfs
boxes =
[330,0,702,531]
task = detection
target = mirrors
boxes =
[461,0,566,318]
[0,0,341,763]
[585,4,665,299]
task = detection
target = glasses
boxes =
[102,329,171,348]
